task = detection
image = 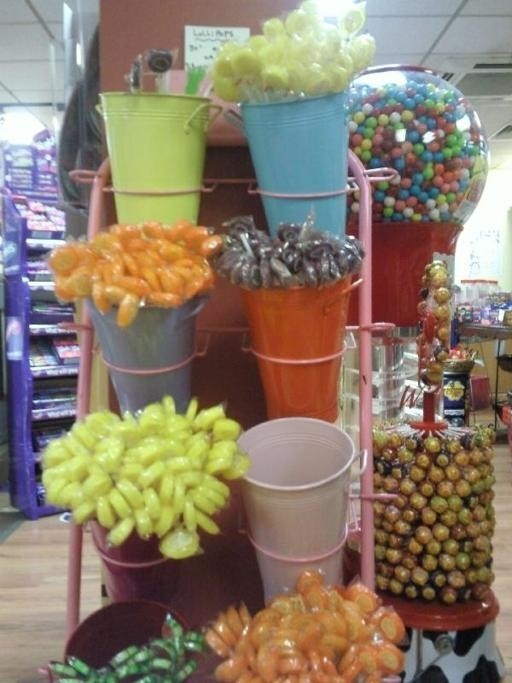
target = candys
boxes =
[211,214,366,293]
[41,395,249,560]
[212,1,376,105]
[345,80,490,225]
[38,562,405,682]
[123,47,212,98]
[349,259,496,607]
[47,219,223,331]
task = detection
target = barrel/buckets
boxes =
[224,88,357,243]
[95,91,221,227]
[233,416,369,609]
[442,373,469,429]
[381,591,500,682]
[237,269,365,421]
[38,600,194,683]
[84,295,211,417]
[84,519,185,605]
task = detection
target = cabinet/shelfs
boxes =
[4,192,83,521]
